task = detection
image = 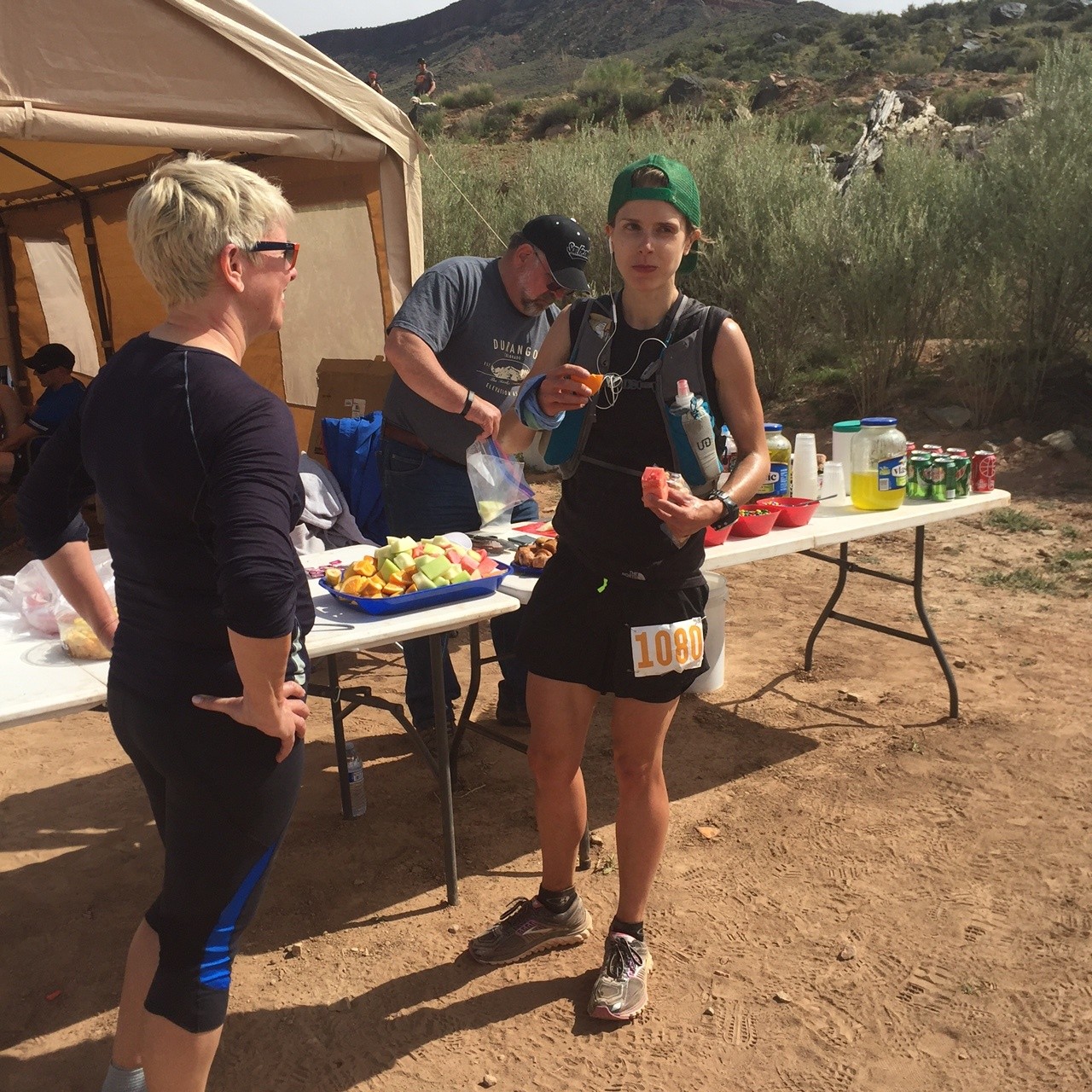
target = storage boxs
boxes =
[307,355,396,469]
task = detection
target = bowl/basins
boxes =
[703,524,733,545]
[755,497,820,526]
[729,504,784,537]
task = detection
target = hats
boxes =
[608,154,700,275]
[521,214,592,296]
[20,343,76,371]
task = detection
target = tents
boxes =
[0,1,425,579]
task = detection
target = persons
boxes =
[0,216,596,763]
[469,152,771,1023]
[16,155,312,1092]
[365,70,382,94]
[408,59,436,127]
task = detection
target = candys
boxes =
[739,502,779,516]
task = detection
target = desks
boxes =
[450,472,1012,791]
[0,543,521,906]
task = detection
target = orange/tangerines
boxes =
[571,373,603,396]
[325,556,418,598]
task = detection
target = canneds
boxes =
[906,441,971,502]
[971,450,996,493]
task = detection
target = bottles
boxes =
[832,420,861,497]
[669,378,704,412]
[750,423,791,504]
[717,424,738,491]
[851,417,908,510]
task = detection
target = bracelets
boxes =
[460,390,474,419]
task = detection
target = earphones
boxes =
[609,236,614,253]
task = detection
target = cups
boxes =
[792,432,820,501]
[821,461,846,503]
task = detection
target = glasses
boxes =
[239,241,299,271]
[527,242,576,296]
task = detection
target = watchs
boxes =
[704,489,740,532]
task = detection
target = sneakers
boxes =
[588,931,654,1019]
[416,717,474,760]
[496,702,531,727]
[468,895,592,965]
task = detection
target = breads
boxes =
[514,534,560,568]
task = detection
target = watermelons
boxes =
[640,466,669,508]
[411,543,499,579]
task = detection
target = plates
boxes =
[510,561,545,574]
